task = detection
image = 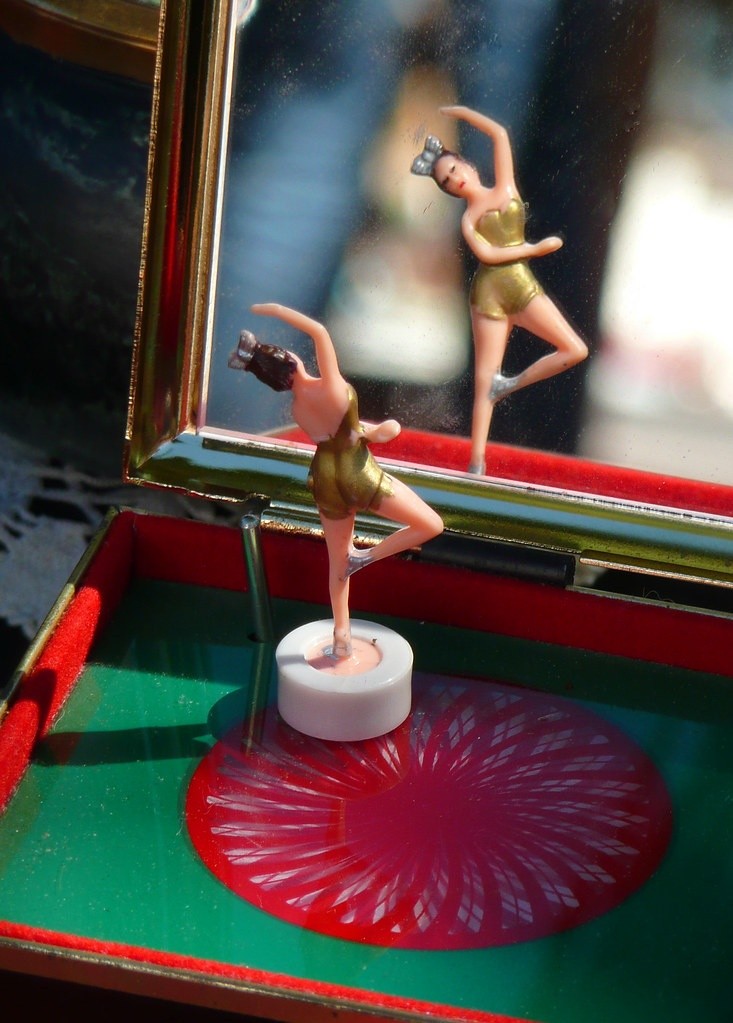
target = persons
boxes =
[225,302,444,661]
[410,105,588,475]
